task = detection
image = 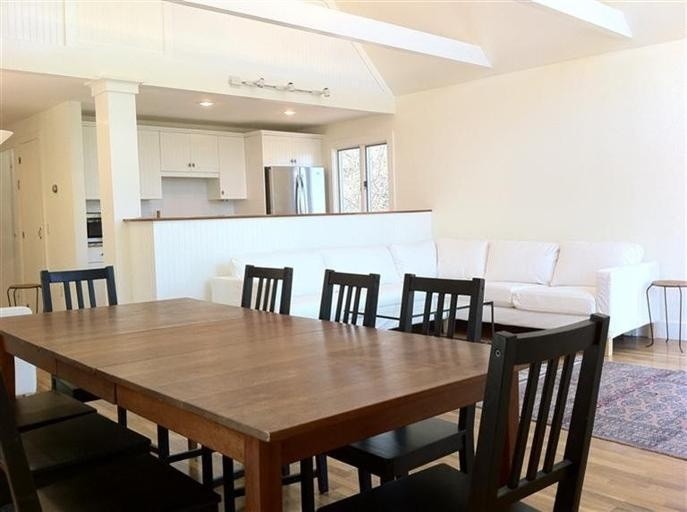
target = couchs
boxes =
[207,240,661,358]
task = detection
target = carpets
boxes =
[475,358,686,460]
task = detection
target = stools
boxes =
[643,279,687,352]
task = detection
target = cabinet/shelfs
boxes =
[82,121,162,201]
[159,128,220,180]
[243,130,327,170]
[206,131,246,202]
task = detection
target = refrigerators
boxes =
[267,165,326,216]
